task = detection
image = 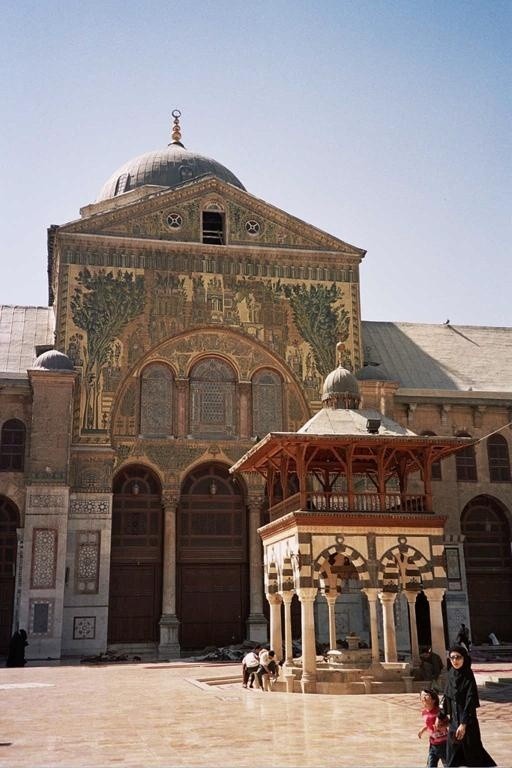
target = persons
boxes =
[417,688,450,766]
[456,624,470,652]
[255,645,269,658]
[434,644,498,767]
[256,650,279,692]
[241,649,260,689]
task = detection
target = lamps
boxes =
[365,418,382,435]
[207,478,217,499]
[131,482,140,500]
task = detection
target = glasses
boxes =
[450,655,461,660]
